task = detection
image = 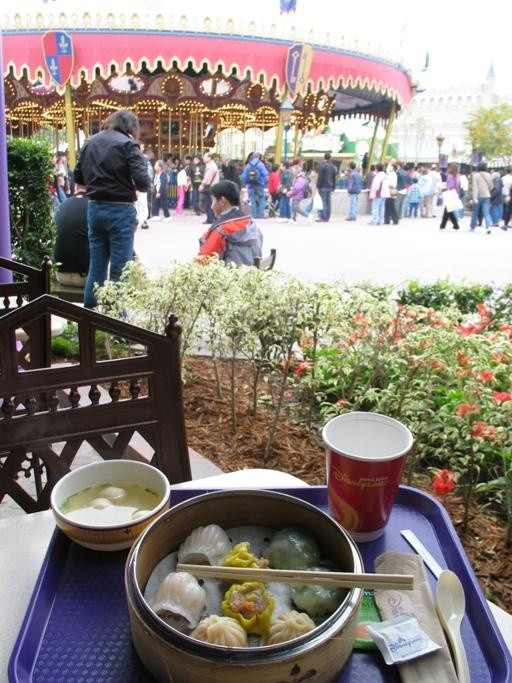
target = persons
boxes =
[195,179,264,266]
[53,184,91,288]
[73,109,151,310]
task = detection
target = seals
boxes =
[147,523,340,648]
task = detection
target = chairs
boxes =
[268,212,327,225]
[439,222,511,234]
[163,217,172,222]
[142,223,148,229]
[150,216,159,221]
[369,214,436,225]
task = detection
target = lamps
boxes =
[254,248,275,272]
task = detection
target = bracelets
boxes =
[49,460,171,555]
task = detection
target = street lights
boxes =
[202,185,210,194]
[390,187,398,197]
[442,188,464,213]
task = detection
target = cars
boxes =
[320,412,413,546]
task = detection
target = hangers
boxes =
[435,571,473,682]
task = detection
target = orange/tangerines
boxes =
[245,184,279,217]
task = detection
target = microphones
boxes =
[51,280,84,302]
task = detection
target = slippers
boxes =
[247,160,263,184]
[304,182,313,199]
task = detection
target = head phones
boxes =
[177,563,415,591]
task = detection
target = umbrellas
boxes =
[203,220,213,224]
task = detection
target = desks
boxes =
[436,133,445,155]
